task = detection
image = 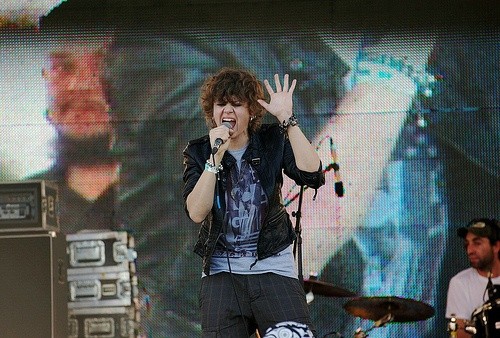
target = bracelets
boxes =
[205,163,219,173]
[206,160,223,170]
[280,116,298,131]
[357,50,436,127]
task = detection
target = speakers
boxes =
[0,231,67,338]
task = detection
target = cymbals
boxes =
[343,296,436,322]
[297,277,357,297]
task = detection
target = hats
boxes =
[457,218,499,238]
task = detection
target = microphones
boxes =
[487,268,493,288]
[329,138,344,198]
[211,122,232,154]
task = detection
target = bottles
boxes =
[447,314,458,338]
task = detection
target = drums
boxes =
[468,299,500,337]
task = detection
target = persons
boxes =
[446,219,500,338]
[182,69,324,338]
[101,28,500,338]
[27,42,141,338]
